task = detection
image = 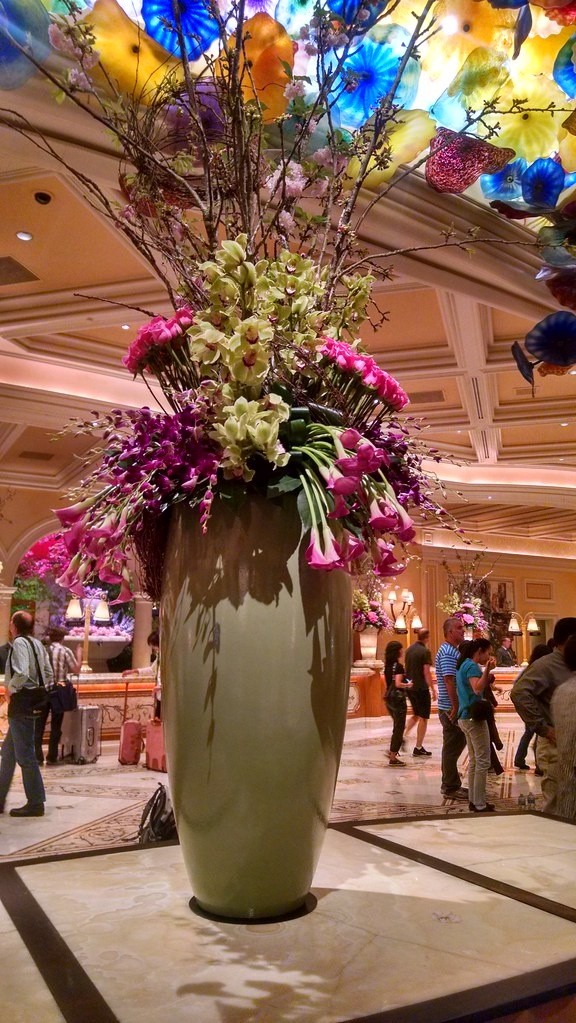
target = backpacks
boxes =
[139,783,175,843]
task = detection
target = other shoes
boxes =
[534,769,543,776]
[469,802,494,811]
[46,757,58,764]
[10,802,44,816]
[35,758,43,765]
[515,763,530,769]
[475,804,496,812]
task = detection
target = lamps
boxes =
[388,589,423,634]
[507,611,539,668]
[91,591,110,622]
[64,591,83,619]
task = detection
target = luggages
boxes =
[118,674,142,765]
[145,690,166,773]
[70,673,99,764]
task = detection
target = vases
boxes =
[464,627,473,641]
[156,470,357,922]
[359,624,378,659]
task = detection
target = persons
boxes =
[457,638,502,812]
[0,610,53,816]
[401,629,437,757]
[509,617,576,820]
[122,630,161,719]
[380,641,413,766]
[35,629,83,765]
[496,638,514,667]
[435,619,468,799]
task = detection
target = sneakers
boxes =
[389,758,405,765]
[440,786,469,794]
[413,747,432,756]
[444,789,468,800]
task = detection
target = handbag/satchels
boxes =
[470,698,493,720]
[11,687,48,713]
[386,662,406,712]
[56,679,77,710]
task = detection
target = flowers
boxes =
[353,589,395,635]
[429,543,503,635]
